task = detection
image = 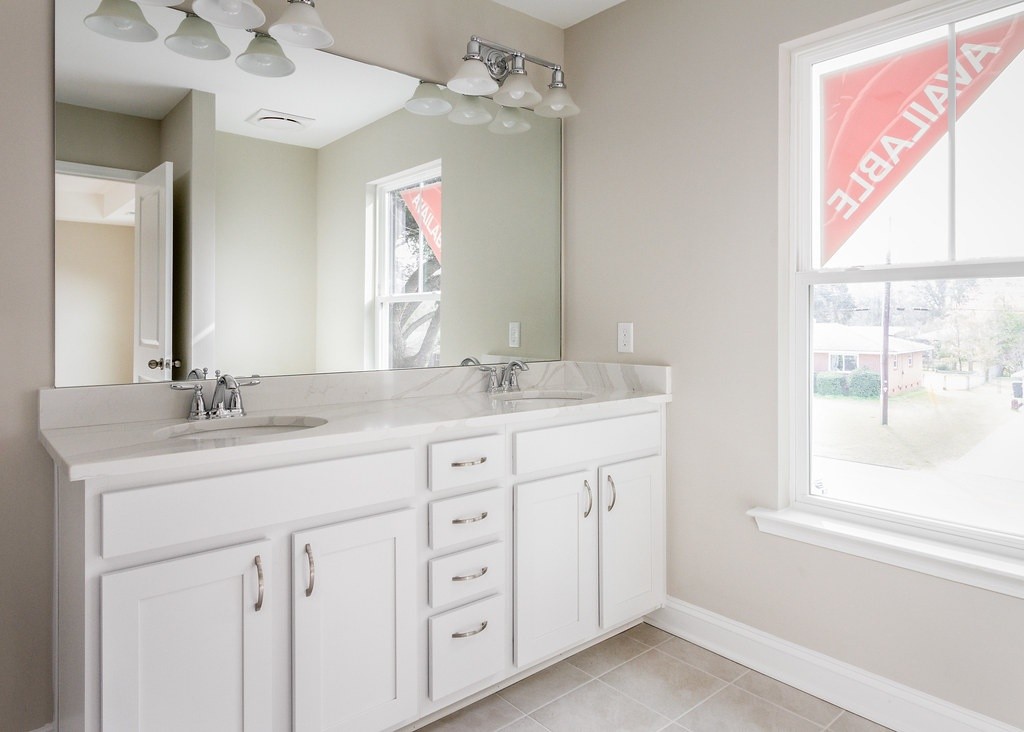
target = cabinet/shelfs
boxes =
[51,402,670,732]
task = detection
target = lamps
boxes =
[133,0,333,49]
[83,0,296,78]
[404,79,533,135]
[446,32,581,118]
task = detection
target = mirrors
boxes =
[55,0,564,388]
